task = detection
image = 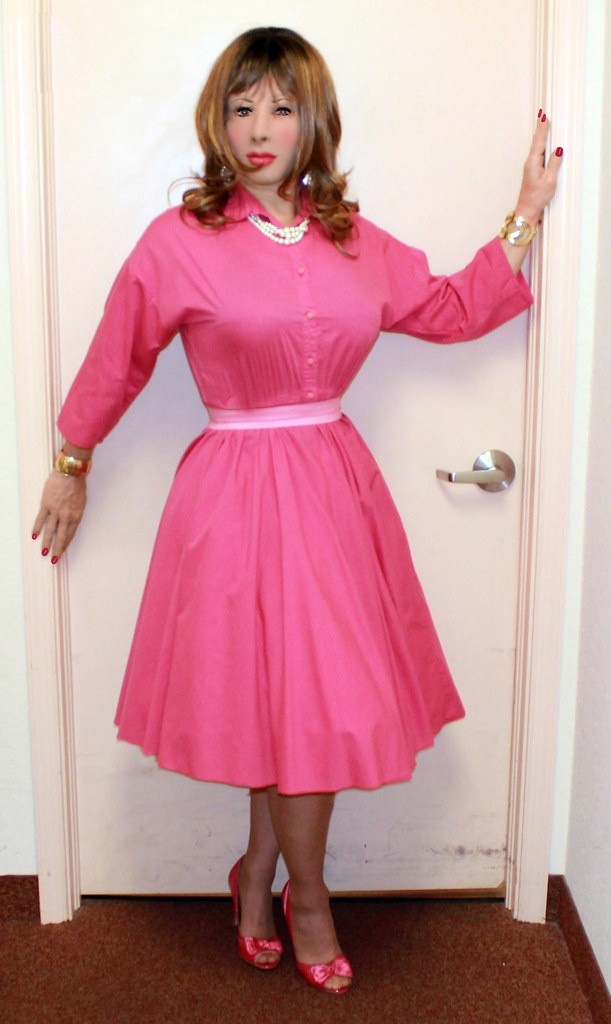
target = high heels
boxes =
[228,854,282,970]
[281,879,353,994]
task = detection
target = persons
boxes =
[30,27,564,995]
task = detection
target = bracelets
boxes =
[52,447,92,479]
[497,211,541,246]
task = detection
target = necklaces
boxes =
[246,214,311,245]
